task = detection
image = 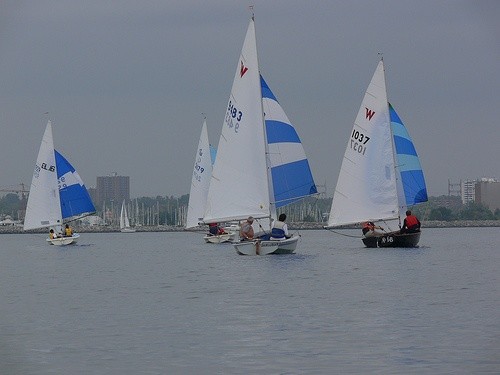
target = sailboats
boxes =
[119,200,136,233]
[197,4,321,256]
[184,112,237,244]
[322,51,429,249]
[22,111,96,247]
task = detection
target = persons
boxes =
[239,216,255,241]
[270,214,288,240]
[400,210,421,234]
[209,223,228,235]
[50,229,57,239]
[362,222,382,235]
[63,224,73,236]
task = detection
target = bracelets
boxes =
[246,237,248,239]
